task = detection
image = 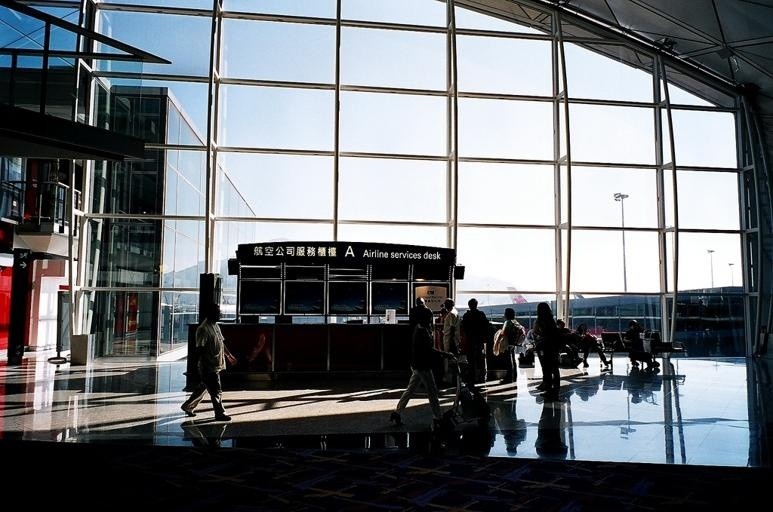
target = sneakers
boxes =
[478,374,517,384]
[181,405,196,417]
[583,358,660,368]
[536,383,559,399]
[215,414,231,421]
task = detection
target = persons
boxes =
[443,299,460,357]
[534,303,559,401]
[391,308,450,431]
[460,299,491,383]
[181,305,237,421]
[535,395,568,460]
[623,320,660,369]
[556,320,583,368]
[460,384,493,457]
[245,330,271,369]
[494,383,526,456]
[409,297,434,331]
[627,369,659,403]
[493,308,519,383]
[574,324,611,367]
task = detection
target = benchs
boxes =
[559,349,615,370]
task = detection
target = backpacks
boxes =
[508,320,526,346]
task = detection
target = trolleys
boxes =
[446,354,494,428]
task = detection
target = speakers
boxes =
[454,265,465,280]
[228,258,239,275]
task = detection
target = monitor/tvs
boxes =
[274,314,292,323]
[348,319,363,325]
[398,319,409,323]
[241,314,259,324]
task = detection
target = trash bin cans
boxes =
[69,334,93,364]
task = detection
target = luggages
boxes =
[452,356,479,421]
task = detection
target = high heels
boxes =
[390,412,406,425]
[431,416,449,432]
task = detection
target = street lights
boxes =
[707,249,714,288]
[612,191,629,291]
[729,263,734,285]
